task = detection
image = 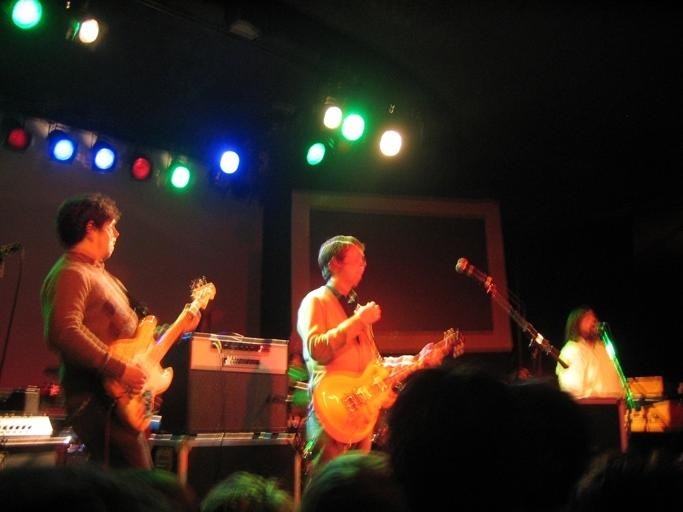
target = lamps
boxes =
[128,153,154,181]
[90,143,119,174]
[46,130,79,165]
[0,121,33,153]
[166,164,193,193]
[1,1,44,33]
[66,16,102,47]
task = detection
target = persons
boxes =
[301,451,402,511]
[39,193,203,472]
[555,305,627,405]
[1,463,75,512]
[197,470,292,512]
[383,365,587,511]
[297,234,442,478]
[574,449,682,512]
[70,469,196,512]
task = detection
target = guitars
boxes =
[314,326,465,442]
[103,276,216,434]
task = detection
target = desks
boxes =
[148,432,304,512]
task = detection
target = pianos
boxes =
[0,414,52,436]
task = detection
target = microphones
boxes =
[454,257,493,288]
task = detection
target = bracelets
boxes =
[94,352,111,380]
